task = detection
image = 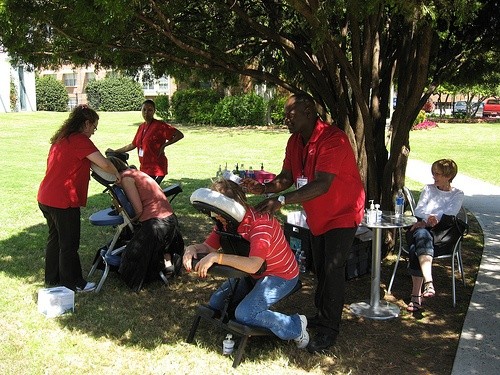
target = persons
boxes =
[37,104,112,293]
[182,180,310,350]
[105,100,184,186]
[240,91,388,352]
[106,156,185,291]
[405,159,464,312]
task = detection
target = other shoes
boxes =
[76,281,97,292]
[306,314,321,329]
[306,333,336,353]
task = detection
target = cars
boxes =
[393,97,397,108]
[451,100,467,113]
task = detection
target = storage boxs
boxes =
[251,169,276,184]
[284,221,312,273]
[38,286,74,318]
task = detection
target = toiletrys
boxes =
[366,199,376,225]
[375,204,382,225]
[395,189,405,215]
[223,333,235,354]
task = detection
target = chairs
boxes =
[387,186,468,308]
[187,230,304,368]
[88,164,182,293]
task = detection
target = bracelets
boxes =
[218,253,223,264]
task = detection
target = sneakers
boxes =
[292,314,310,349]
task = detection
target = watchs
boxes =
[278,195,286,205]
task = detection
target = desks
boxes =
[348,211,417,320]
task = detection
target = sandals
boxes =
[406,294,423,312]
[423,281,435,299]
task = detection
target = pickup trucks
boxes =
[483,97,500,118]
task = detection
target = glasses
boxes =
[90,121,97,132]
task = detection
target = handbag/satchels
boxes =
[429,213,470,256]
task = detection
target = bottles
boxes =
[240,164,254,179]
[216,162,240,178]
[395,188,404,218]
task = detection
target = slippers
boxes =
[163,253,182,287]
